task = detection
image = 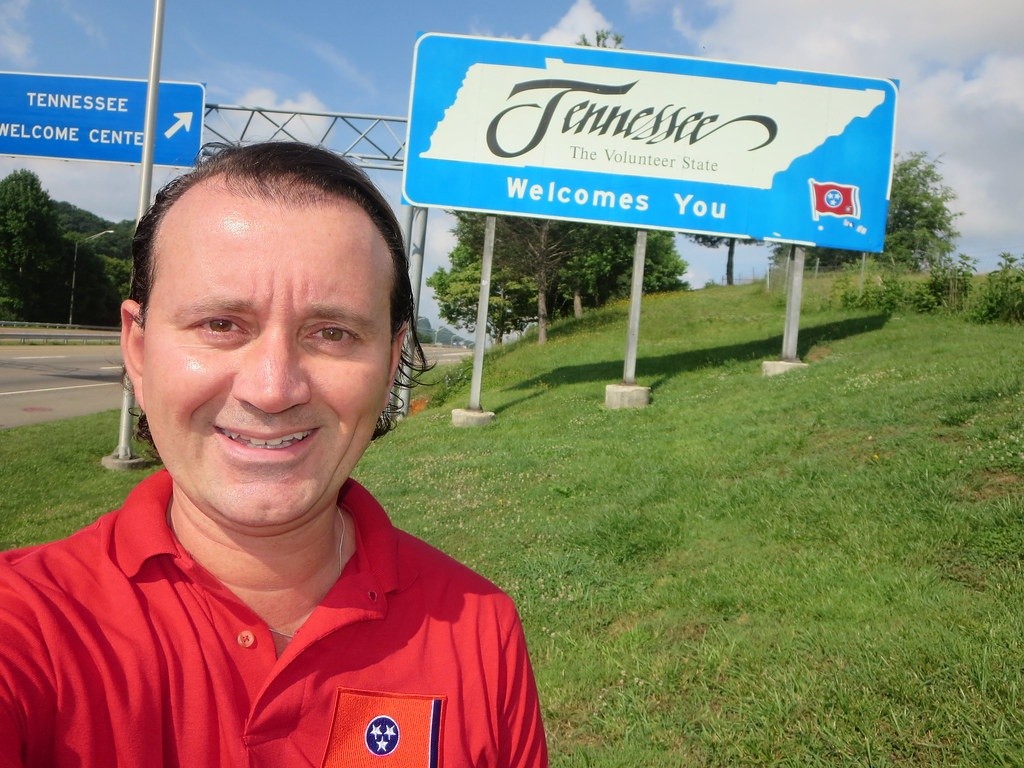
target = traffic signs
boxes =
[0,70,205,171]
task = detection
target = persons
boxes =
[0,141,547,768]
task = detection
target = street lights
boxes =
[68,228,116,329]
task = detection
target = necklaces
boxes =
[266,505,346,638]
